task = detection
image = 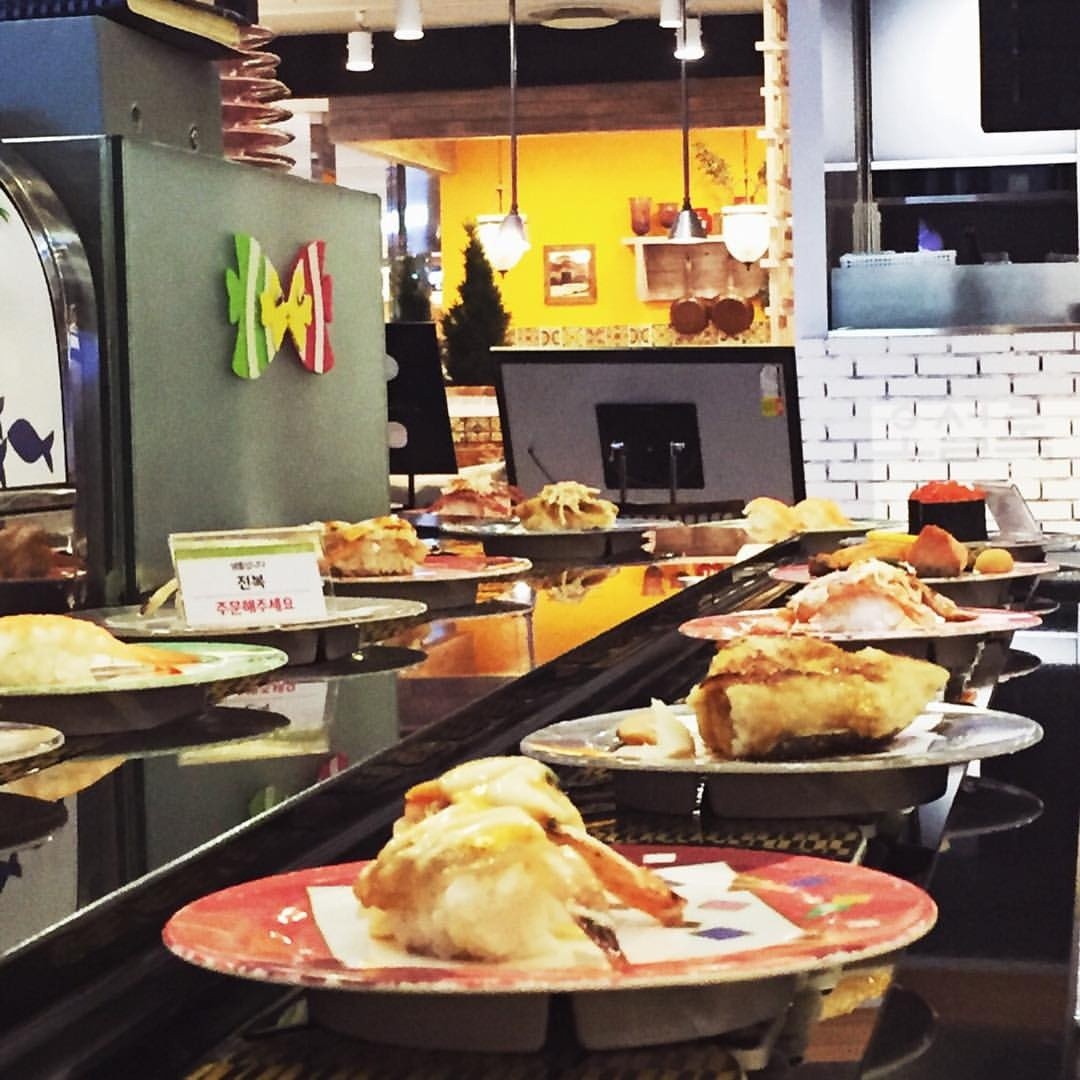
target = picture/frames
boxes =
[544,245,595,306]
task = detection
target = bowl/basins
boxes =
[908,496,989,543]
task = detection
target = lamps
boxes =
[666,2,712,244]
[392,0,426,43]
[343,8,377,74]
[474,2,530,278]
[665,3,701,59]
[720,126,770,271]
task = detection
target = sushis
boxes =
[0,615,201,687]
[402,472,526,520]
[741,496,851,529]
[907,480,992,543]
[354,755,687,971]
[777,561,978,631]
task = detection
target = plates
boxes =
[63,595,428,639]
[1,643,290,696]
[679,607,1043,643]
[768,561,1059,583]
[518,701,1043,774]
[322,556,532,583]
[163,843,939,995]
[441,517,685,535]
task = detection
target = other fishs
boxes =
[289,240,336,375]
[226,232,288,380]
